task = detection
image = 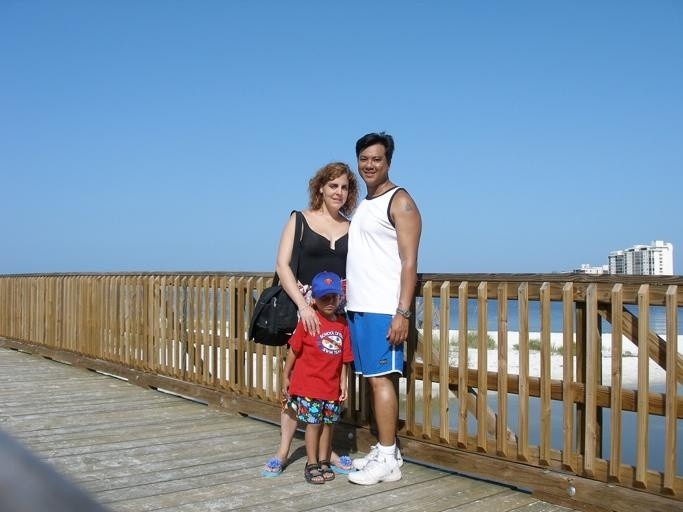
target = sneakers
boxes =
[347,456,404,487]
[350,442,409,470]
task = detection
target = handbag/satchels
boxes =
[246,283,298,347]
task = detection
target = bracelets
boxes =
[298,304,309,315]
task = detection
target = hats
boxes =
[311,269,345,301]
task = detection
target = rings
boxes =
[399,335,404,339]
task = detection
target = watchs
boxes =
[396,308,411,320]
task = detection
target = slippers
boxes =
[330,454,360,476]
[260,456,286,479]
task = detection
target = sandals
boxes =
[318,461,337,483]
[304,460,325,486]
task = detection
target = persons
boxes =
[344,130,422,487]
[279,268,356,486]
[261,160,364,478]
